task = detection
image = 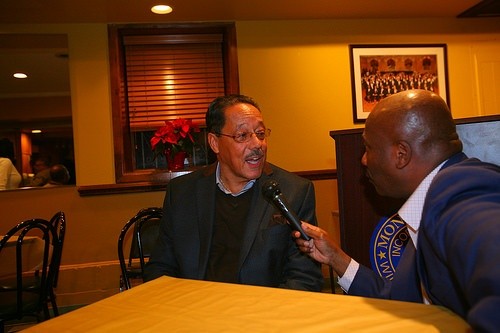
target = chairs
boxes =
[41,210,66,320]
[114,207,167,289]
[0,218,61,332]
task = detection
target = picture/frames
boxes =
[348,43,451,124]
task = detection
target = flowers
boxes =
[151,117,201,149]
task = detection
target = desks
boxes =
[18,274,475,333]
[327,113,500,273]
[0,235,46,313]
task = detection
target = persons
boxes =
[143,94,324,291]
[44,164,70,187]
[292,89,500,333]
[362,70,434,104]
[0,138,22,190]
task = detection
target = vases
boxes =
[165,140,186,171]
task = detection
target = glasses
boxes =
[218,129,272,143]
